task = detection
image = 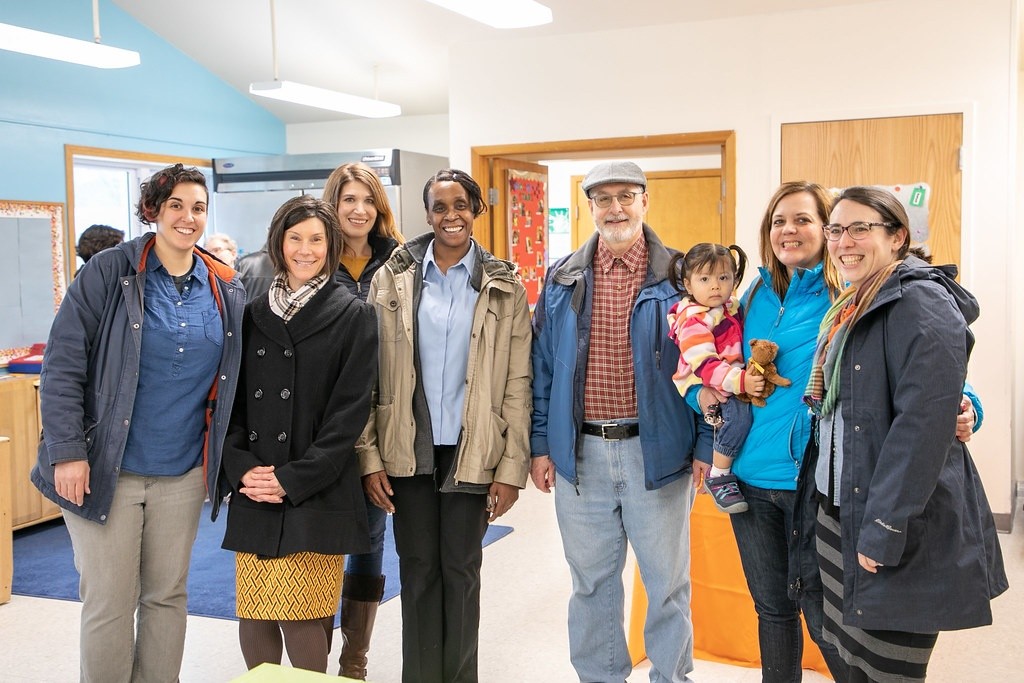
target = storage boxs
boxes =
[7,352,45,374]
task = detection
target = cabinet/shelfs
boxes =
[0,374,62,531]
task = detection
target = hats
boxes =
[581,160,647,195]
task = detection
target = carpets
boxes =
[11,496,514,629]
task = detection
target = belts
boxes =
[579,420,639,442]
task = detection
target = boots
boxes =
[317,614,334,654]
[339,571,386,680]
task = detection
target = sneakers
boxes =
[703,465,748,514]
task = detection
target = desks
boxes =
[626,488,833,679]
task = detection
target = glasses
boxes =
[823,222,903,242]
[590,191,645,209]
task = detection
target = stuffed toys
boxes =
[736,338,792,407]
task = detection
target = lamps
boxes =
[248,0,401,119]
[427,1,554,29]
[0,0,141,70]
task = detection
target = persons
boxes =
[666,242,765,514]
[220,162,407,683]
[529,161,714,507]
[781,186,1010,683]
[73,224,125,278]
[31,163,246,683]
[204,228,282,304]
[354,169,534,683]
[730,181,984,683]
